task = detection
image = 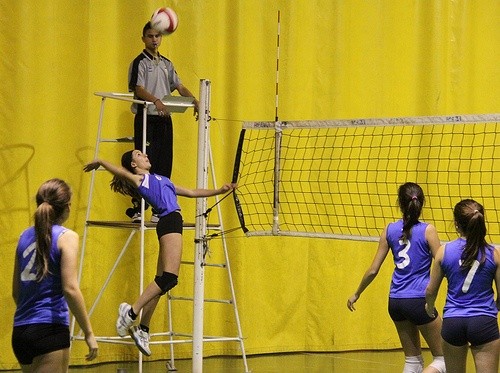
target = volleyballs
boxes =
[151,7,178,33]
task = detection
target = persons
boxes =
[80,148,241,358]
[423,197,499,373]
[124,20,201,224]
[345,181,447,372]
[10,175,99,373]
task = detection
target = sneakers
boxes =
[132,212,142,222]
[129,324,151,356]
[151,215,159,222]
[115,302,137,337]
